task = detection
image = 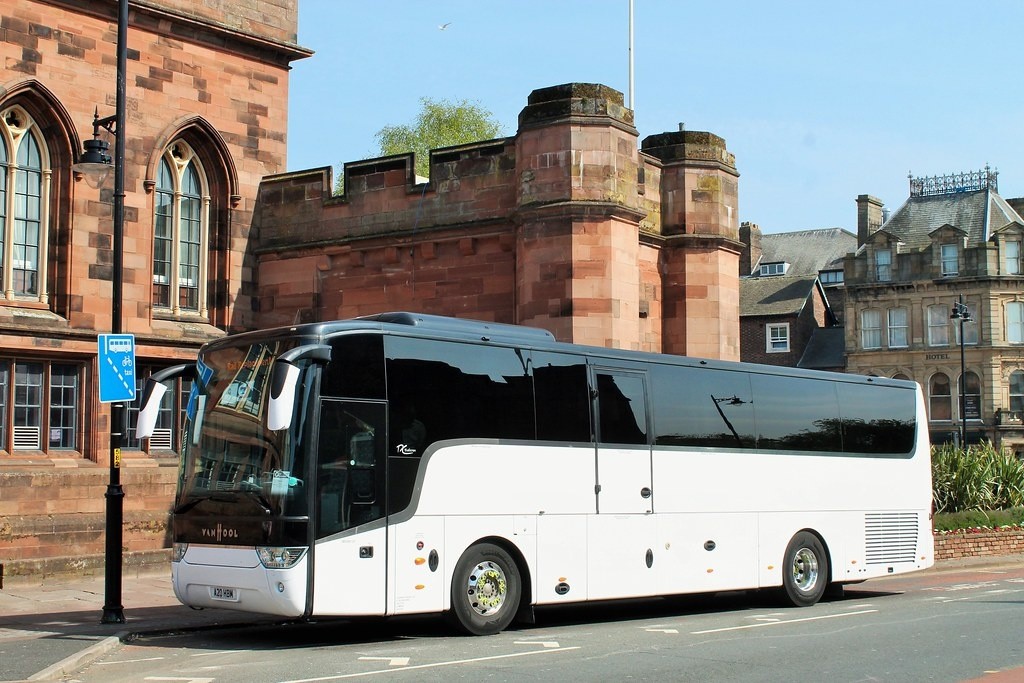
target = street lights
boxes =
[949,302,973,451]
[70,105,127,625]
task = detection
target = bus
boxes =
[135,313,936,636]
